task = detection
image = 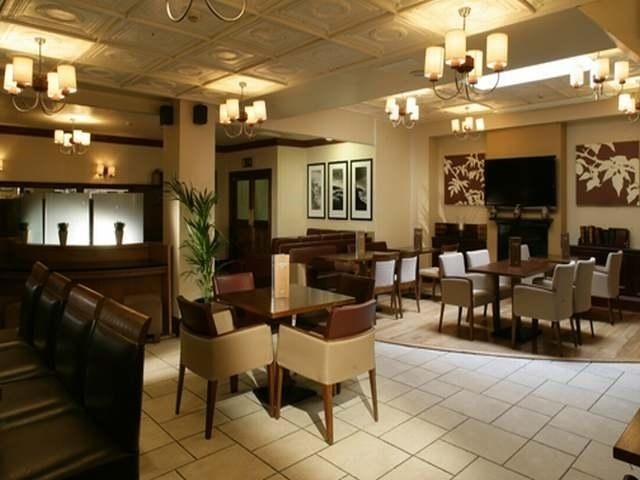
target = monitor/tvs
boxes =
[484,154,559,211]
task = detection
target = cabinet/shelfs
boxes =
[490,216,553,263]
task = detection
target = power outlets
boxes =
[423,7,509,100]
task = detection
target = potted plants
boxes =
[114,222,125,245]
[58,222,68,245]
[20,221,29,243]
[159,170,229,304]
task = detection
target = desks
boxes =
[613,408,640,468]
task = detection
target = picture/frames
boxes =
[307,158,372,221]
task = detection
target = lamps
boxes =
[616,94,640,124]
[2,37,78,115]
[219,82,268,139]
[450,107,486,142]
[570,46,629,102]
[384,95,419,130]
[54,120,91,158]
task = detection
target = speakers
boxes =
[158,104,174,126]
[192,104,209,125]
